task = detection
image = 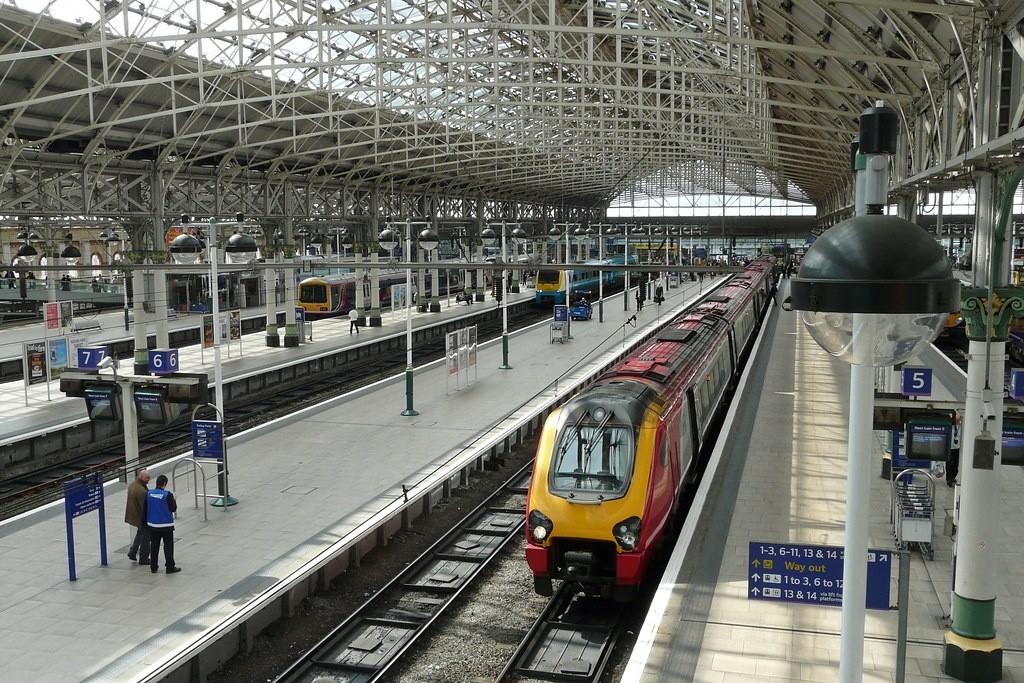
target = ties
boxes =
[954,425,958,444]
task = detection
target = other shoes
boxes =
[151,568,156,573]
[139,559,151,565]
[128,552,137,560]
[166,566,181,574]
[947,480,952,487]
[952,479,956,483]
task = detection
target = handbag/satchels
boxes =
[653,296,658,302]
[661,295,664,301]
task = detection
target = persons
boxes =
[781,259,796,279]
[462,292,474,306]
[91,273,118,294]
[580,297,587,305]
[274,281,286,291]
[124,469,153,565]
[140,475,181,574]
[770,283,777,306]
[5,271,71,291]
[348,306,360,336]
[379,288,383,312]
[201,289,236,310]
[508,269,527,293]
[656,282,663,305]
[681,256,752,283]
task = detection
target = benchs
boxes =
[167,309,177,318]
[72,318,102,334]
[123,312,135,328]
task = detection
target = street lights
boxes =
[584,220,615,324]
[550,220,587,340]
[169,216,258,507]
[613,222,709,311]
[378,217,440,418]
[779,212,962,683]
[481,220,526,369]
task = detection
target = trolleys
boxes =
[892,484,935,561]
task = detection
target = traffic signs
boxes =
[749,541,891,611]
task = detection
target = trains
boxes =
[524,252,778,610]
[535,255,639,309]
[297,252,533,316]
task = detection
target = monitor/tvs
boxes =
[83,389,120,423]
[905,419,953,462]
[133,392,171,426]
[1001,419,1024,464]
